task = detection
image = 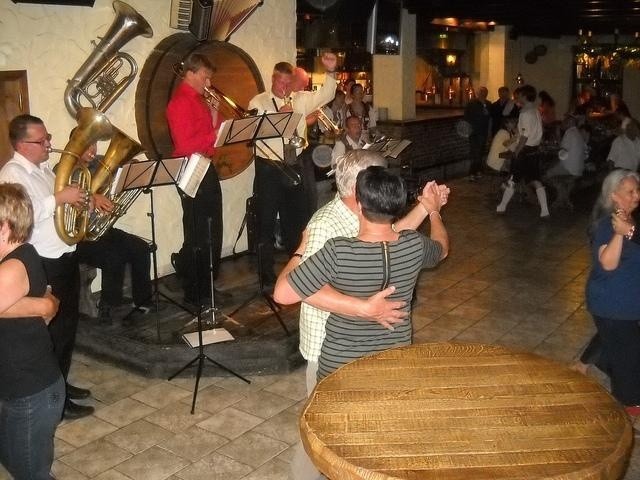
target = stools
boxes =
[79,233,157,318]
[481,167,512,211]
[547,175,577,215]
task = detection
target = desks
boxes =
[298,341,632,480]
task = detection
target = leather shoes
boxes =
[196,300,224,310]
[96,307,113,327]
[531,214,551,225]
[258,266,278,287]
[63,400,95,420]
[66,381,91,400]
[137,298,167,313]
[208,289,232,297]
[488,207,504,217]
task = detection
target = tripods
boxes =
[167,210,251,414]
[228,141,291,337]
[122,189,199,345]
[171,215,248,332]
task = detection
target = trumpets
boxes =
[319,107,345,135]
[282,91,306,149]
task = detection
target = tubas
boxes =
[47,107,114,245]
[63,0,153,123]
[80,122,145,243]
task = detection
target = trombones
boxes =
[170,57,302,185]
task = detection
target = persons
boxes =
[247,50,378,285]
[0,113,97,420]
[51,125,169,327]
[165,52,233,306]
[297,148,450,398]
[0,284,61,326]
[569,168,640,436]
[0,181,67,480]
[272,165,450,480]
[464,83,640,223]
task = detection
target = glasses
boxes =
[22,133,52,147]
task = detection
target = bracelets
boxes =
[428,210,443,222]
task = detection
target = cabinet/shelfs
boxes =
[297,47,372,96]
[571,43,623,112]
